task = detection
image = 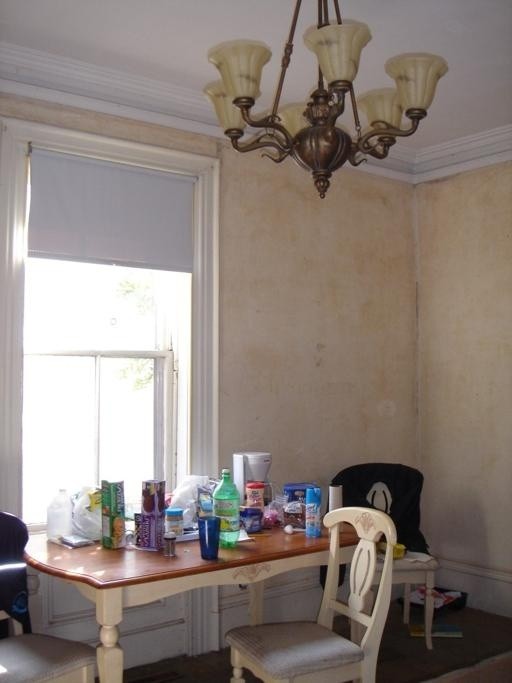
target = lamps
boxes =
[203,0,449,199]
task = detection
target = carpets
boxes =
[120,598,512,683]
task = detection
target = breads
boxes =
[284,503,306,529]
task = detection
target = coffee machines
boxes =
[232,450,276,504]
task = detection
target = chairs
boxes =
[0,512,96,682]
[224,506,398,682]
[326,463,439,650]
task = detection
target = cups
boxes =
[198,515,222,560]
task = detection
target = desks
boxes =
[21,518,381,683]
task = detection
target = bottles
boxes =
[164,506,183,536]
[162,530,178,557]
[245,481,265,511]
[211,467,242,548]
[46,489,74,543]
[306,486,322,537]
